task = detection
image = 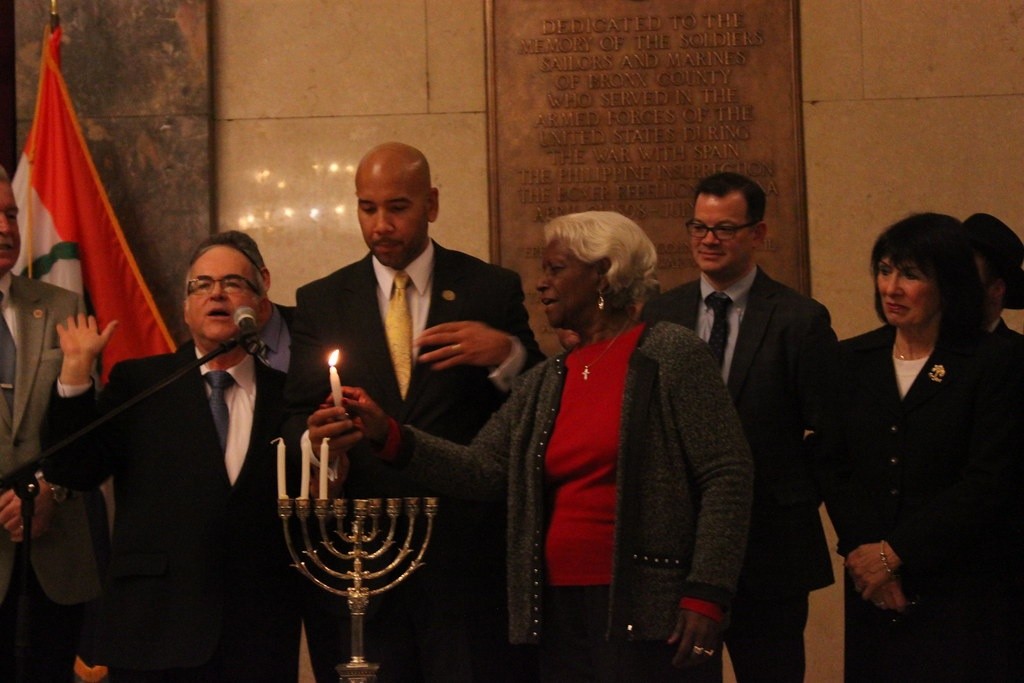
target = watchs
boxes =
[40,473,71,508]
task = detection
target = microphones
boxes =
[232,305,257,332]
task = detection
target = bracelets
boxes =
[880,540,894,575]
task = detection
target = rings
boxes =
[877,602,885,606]
[452,342,462,355]
[704,650,715,656]
[693,646,704,654]
[20,524,24,531]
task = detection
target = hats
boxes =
[961,213,1024,309]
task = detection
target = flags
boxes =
[9,20,175,683]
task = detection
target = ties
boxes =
[385,272,413,400]
[0,309,15,417]
[702,293,732,370]
[204,370,234,456]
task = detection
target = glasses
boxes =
[187,278,257,296]
[684,218,757,241]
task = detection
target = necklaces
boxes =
[574,319,630,380]
[894,341,934,359]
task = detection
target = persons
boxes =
[0,139,1024,683]
